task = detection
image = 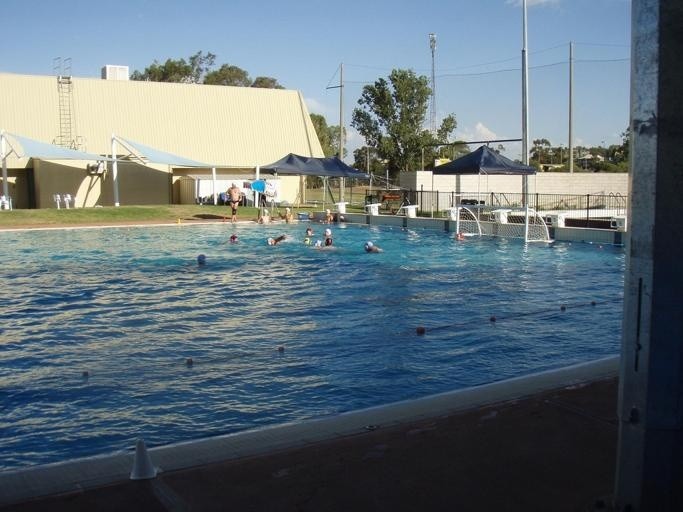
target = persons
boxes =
[323,228,331,236]
[278,208,292,224]
[270,189,278,212]
[257,209,270,224]
[325,238,331,246]
[314,240,321,246]
[304,237,311,245]
[196,254,205,263]
[456,232,464,240]
[266,236,281,245]
[226,182,242,223]
[305,228,312,235]
[281,234,288,239]
[230,235,237,242]
[318,209,333,223]
[363,241,379,251]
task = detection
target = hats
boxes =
[197,253,206,262]
[364,240,372,251]
[228,208,335,246]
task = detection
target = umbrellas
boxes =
[250,179,273,196]
